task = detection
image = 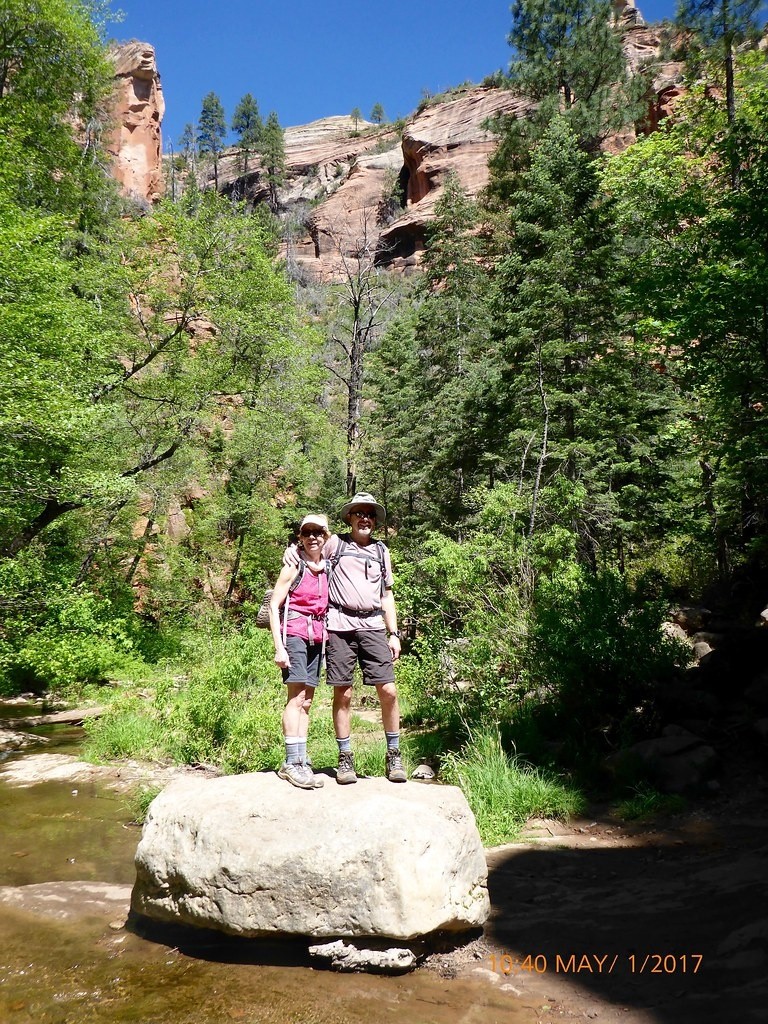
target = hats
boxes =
[339,492,386,530]
[300,514,329,532]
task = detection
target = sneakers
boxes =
[302,765,324,788]
[277,760,314,788]
[386,749,407,782]
[336,751,358,784]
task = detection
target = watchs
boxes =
[389,630,400,638]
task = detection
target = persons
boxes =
[267,513,332,789]
[281,490,408,784]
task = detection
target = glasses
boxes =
[301,529,324,537]
[350,511,376,519]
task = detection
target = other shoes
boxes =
[256,590,273,628]
